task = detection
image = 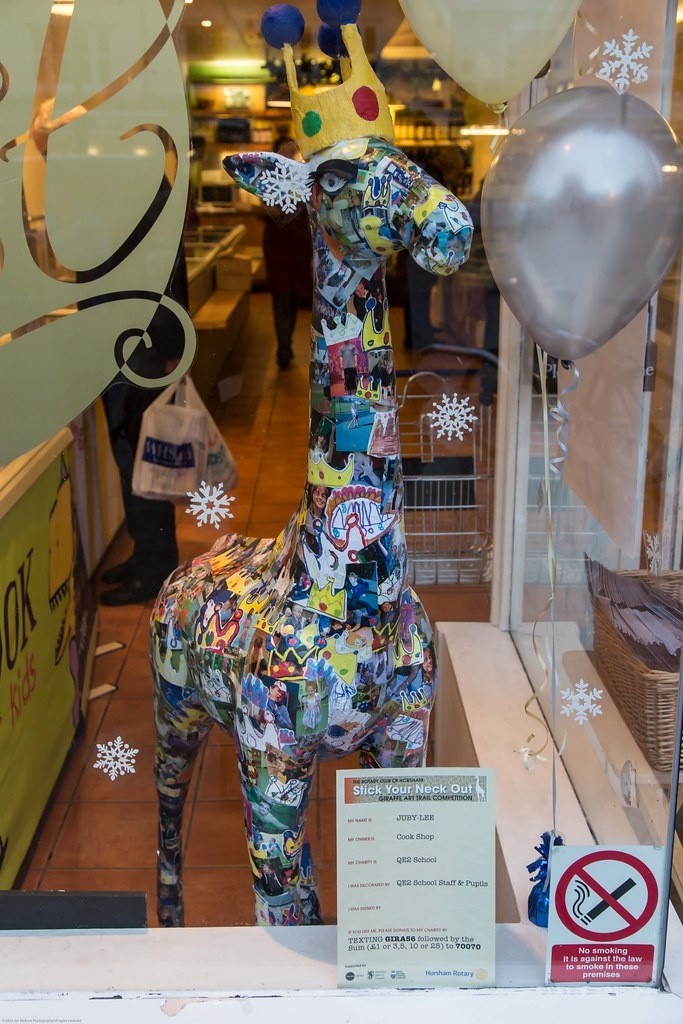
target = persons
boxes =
[266,136,341,369]
[395,145,469,353]
[33,97,190,607]
[314,249,341,256]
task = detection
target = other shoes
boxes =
[404,337,447,352]
[100,572,162,608]
[100,558,135,585]
[277,352,296,369]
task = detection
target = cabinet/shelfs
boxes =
[191,105,471,219]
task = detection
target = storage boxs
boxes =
[212,252,252,290]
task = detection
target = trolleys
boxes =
[381,364,501,590]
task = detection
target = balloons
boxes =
[481,86,683,370]
[399,0,582,114]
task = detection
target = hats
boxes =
[272,681,288,696]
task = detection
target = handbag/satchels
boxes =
[179,372,239,499]
[131,372,207,502]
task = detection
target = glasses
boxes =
[313,490,327,500]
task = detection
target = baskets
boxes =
[592,569,683,774]
